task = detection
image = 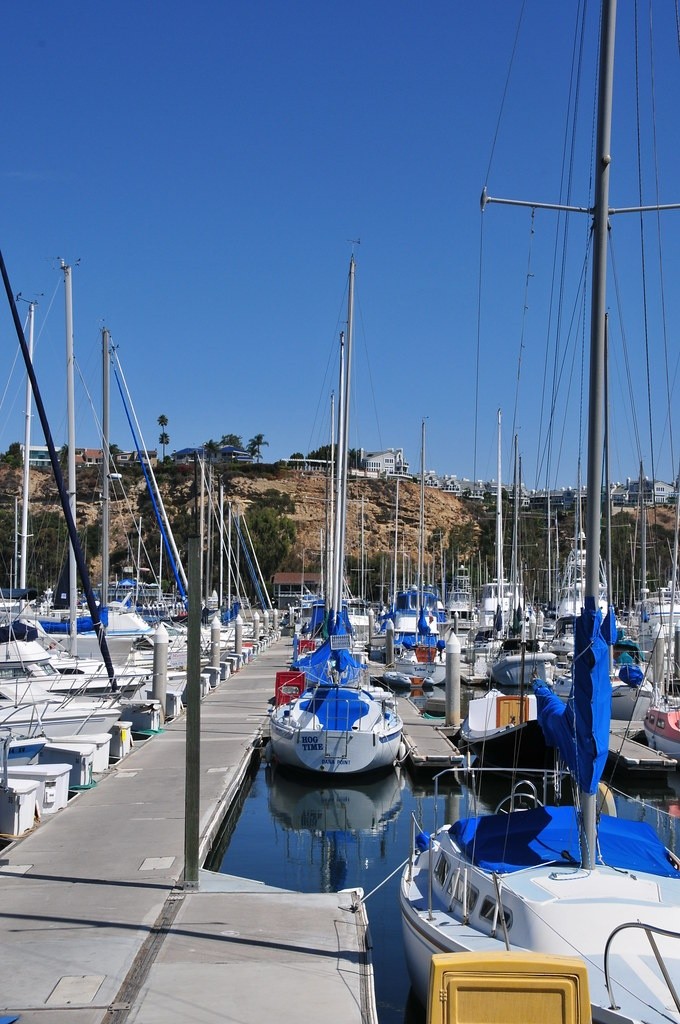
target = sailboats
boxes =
[0,55,680,1024]
